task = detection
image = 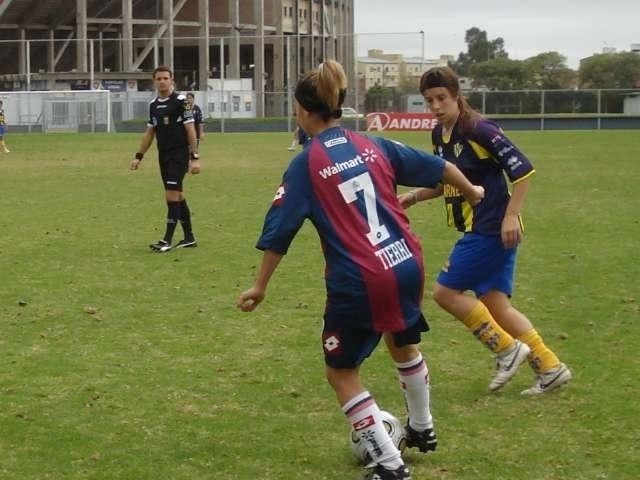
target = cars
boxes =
[341,107,366,119]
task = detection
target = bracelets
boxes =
[136,152,143,161]
[410,187,419,206]
[191,152,200,159]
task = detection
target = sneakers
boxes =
[0,144,10,154]
[288,146,296,151]
[489,339,531,391]
[520,362,572,395]
[365,464,412,480]
[404,417,437,452]
[173,239,197,248]
[149,240,172,252]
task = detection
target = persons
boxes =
[286,125,300,153]
[235,60,484,479]
[0,100,10,153]
[397,66,573,397]
[128,66,201,253]
[186,93,204,146]
[296,122,312,151]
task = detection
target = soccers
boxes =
[350,412,407,466]
